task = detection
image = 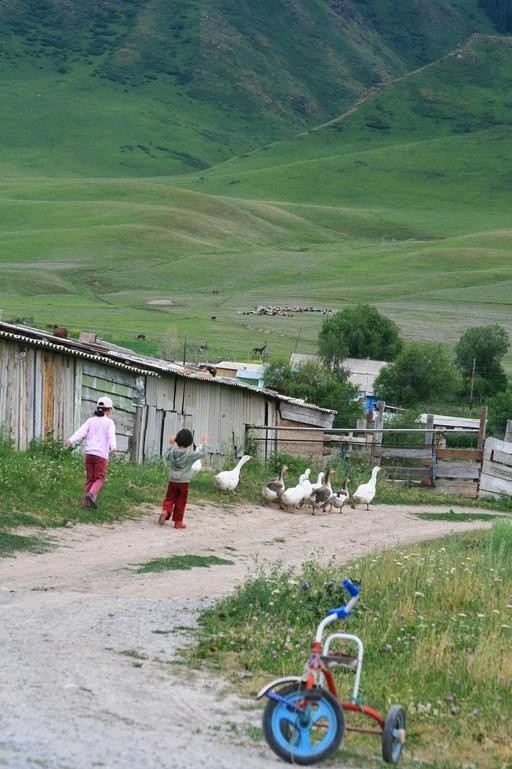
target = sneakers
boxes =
[85,493,97,509]
[174,520,187,529]
[159,510,167,526]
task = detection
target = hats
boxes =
[96,396,114,411]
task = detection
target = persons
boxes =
[156,427,211,530]
[62,395,117,508]
[434,433,446,450]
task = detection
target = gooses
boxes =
[351,466,383,511]
[261,464,351,515]
[189,444,202,479]
[212,455,254,496]
